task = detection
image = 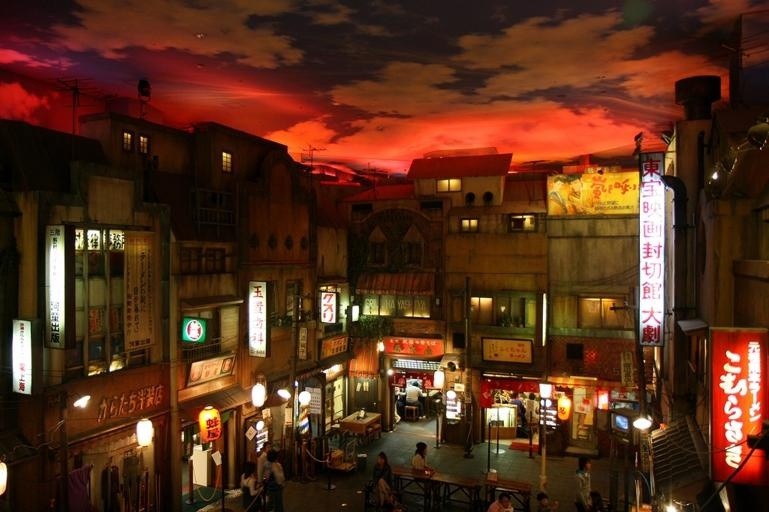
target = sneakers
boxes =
[419,415,426,419]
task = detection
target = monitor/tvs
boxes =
[610,409,640,438]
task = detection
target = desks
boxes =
[388,465,534,512]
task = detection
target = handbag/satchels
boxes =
[262,471,275,487]
[242,485,261,511]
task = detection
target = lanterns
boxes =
[252,384,265,406]
[434,370,444,388]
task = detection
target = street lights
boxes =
[538,375,556,494]
[633,408,661,512]
[55,388,93,512]
[278,379,311,478]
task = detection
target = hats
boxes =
[416,442,426,449]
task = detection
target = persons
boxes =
[261,450,286,512]
[256,441,273,512]
[525,393,539,424]
[535,493,559,512]
[509,392,525,425]
[411,442,432,470]
[241,462,262,511]
[589,491,604,512]
[574,456,592,512]
[405,381,426,420]
[488,492,514,512]
[366,451,392,508]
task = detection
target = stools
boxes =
[396,390,442,421]
[329,409,382,451]
